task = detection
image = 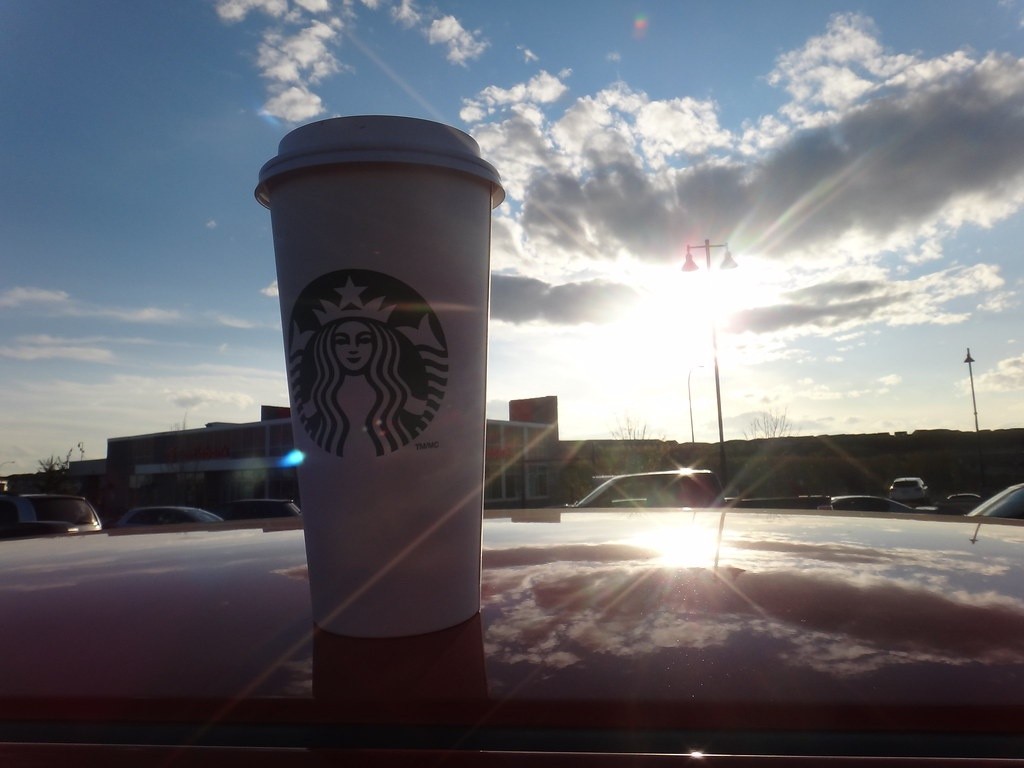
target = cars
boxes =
[889,478,930,506]
[218,498,301,522]
[113,506,225,526]
[574,470,727,508]
[937,494,984,514]
[968,483,1024,519]
[831,495,940,515]
[14,493,101,536]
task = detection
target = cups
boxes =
[255,112,509,640]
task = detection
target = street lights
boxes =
[684,240,738,484]
[964,347,980,432]
